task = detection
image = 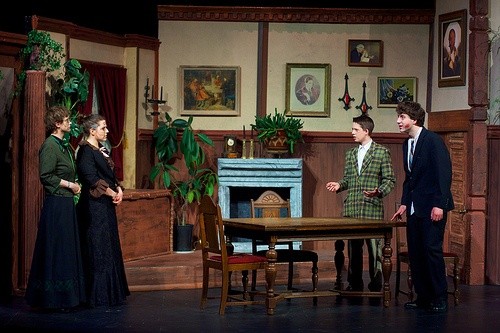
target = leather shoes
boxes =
[405,300,425,310]
[430,302,448,313]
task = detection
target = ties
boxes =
[409,140,414,171]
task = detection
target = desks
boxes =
[216,217,407,315]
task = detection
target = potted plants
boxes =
[150,112,220,252]
[250,108,305,155]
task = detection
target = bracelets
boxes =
[68,182,70,187]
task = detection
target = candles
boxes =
[243,125,245,139]
[251,127,253,139]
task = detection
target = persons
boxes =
[76,113,131,312]
[390,101,455,315]
[326,115,396,306]
[24,104,85,313]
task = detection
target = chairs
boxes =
[250,190,318,306]
[198,194,267,315]
[394,202,459,306]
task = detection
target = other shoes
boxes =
[52,307,74,313]
[368,281,382,292]
[346,284,364,291]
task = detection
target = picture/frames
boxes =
[285,63,330,117]
[180,65,241,115]
[438,9,467,87]
[377,77,417,108]
[348,39,383,67]
